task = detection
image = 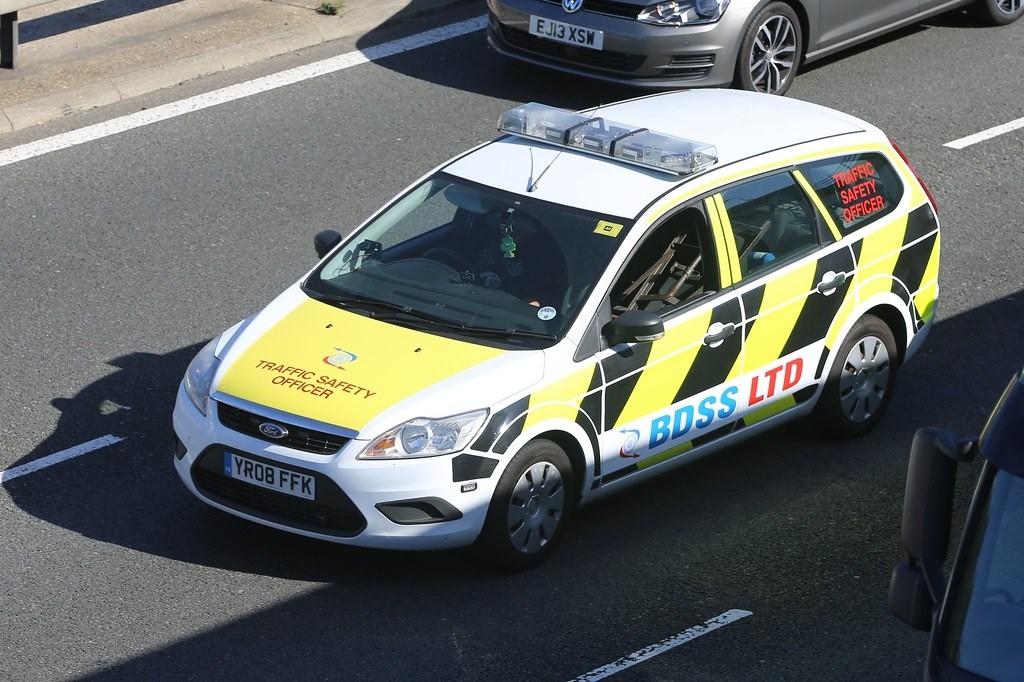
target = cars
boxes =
[172,86,942,577]
[486,0,1024,99]
